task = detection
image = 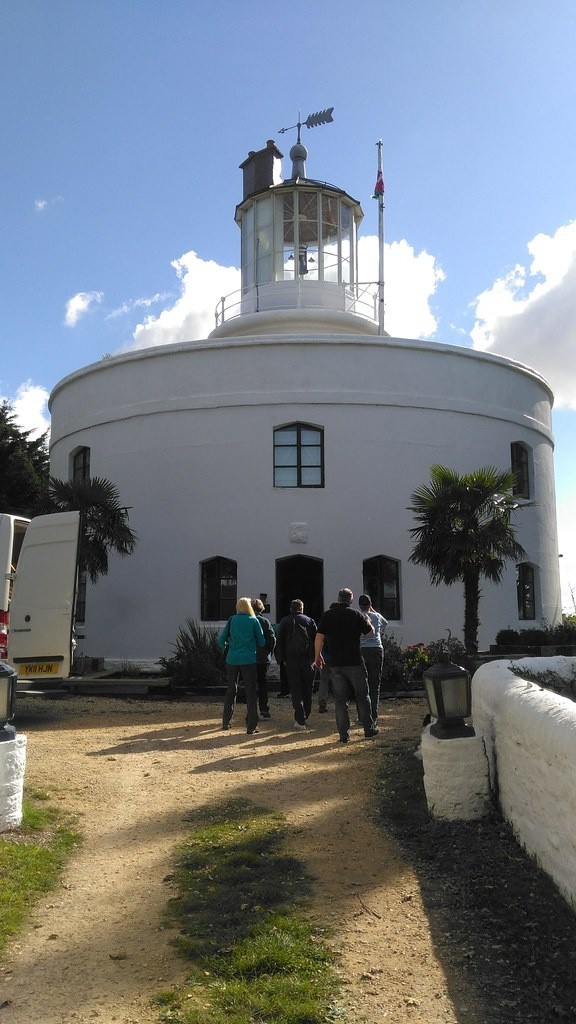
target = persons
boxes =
[219,597,265,734]
[314,588,379,742]
[251,594,388,725]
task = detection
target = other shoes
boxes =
[249,727,260,733]
[321,709,328,713]
[226,723,233,729]
[260,711,271,719]
[294,722,306,730]
[346,730,352,737]
[365,726,379,739]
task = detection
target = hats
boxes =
[290,599,304,614]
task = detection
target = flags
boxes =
[371,166,385,199]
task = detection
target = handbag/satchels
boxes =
[221,615,232,660]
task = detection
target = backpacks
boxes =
[284,614,311,660]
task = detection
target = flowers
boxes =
[400,642,430,684]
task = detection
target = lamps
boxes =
[422,646,475,740]
[0,662,19,742]
